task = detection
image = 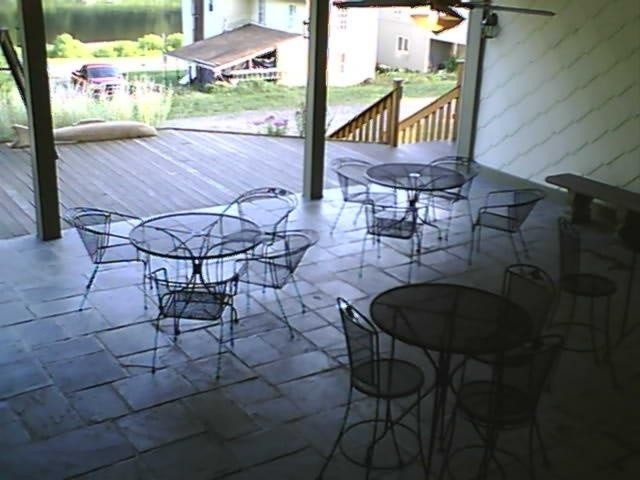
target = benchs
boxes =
[544,171,639,241]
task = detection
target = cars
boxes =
[71,63,124,99]
